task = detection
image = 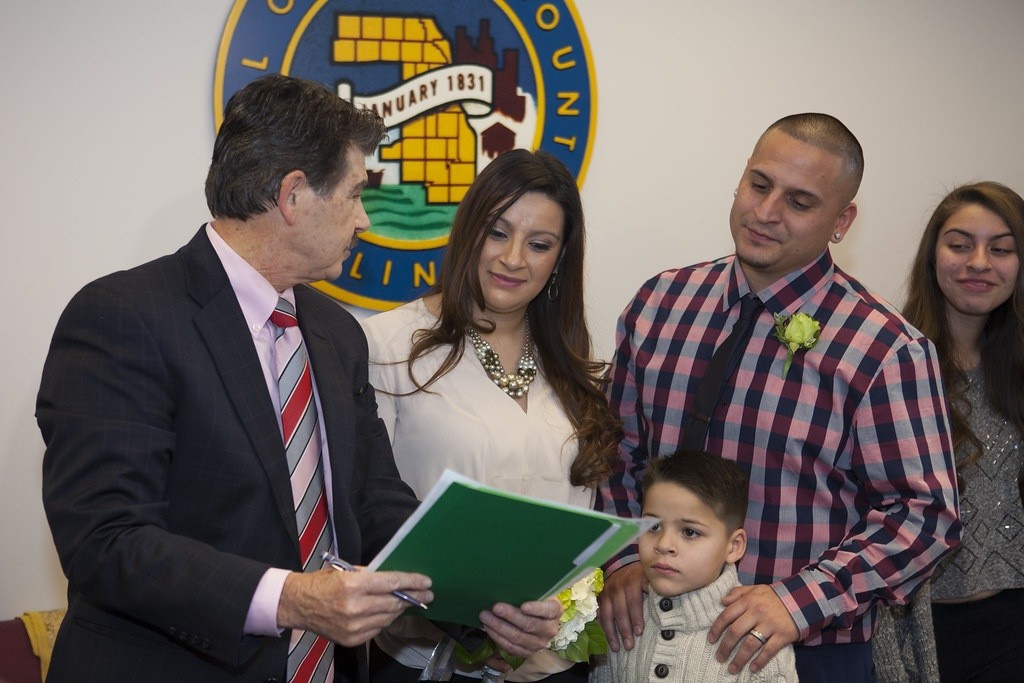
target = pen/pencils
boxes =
[322,552,429,610]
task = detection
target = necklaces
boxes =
[466,311,537,398]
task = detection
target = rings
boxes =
[750,628,767,644]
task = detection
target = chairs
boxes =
[0,609,66,683]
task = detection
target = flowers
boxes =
[455,575,611,670]
[771,312,822,381]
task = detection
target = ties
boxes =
[676,296,764,452]
[270,296,335,683]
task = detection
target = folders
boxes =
[372,479,640,653]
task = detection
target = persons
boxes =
[34,74,563,682]
[589,452,799,683]
[901,181,1024,682]
[598,113,964,681]
[357,151,624,683]
